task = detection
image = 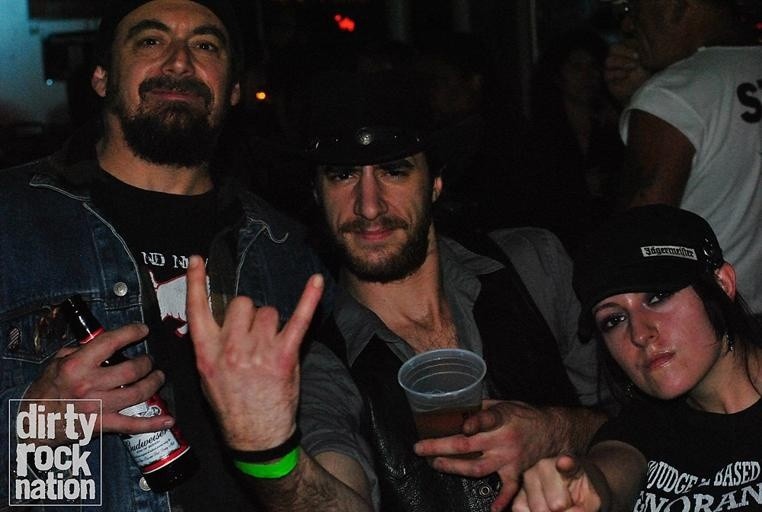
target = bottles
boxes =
[60,293,200,492]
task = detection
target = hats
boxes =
[96,1,240,35]
[303,94,440,165]
[578,204,722,343]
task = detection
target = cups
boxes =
[399,350,488,438]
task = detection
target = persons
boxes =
[0,2,762,512]
